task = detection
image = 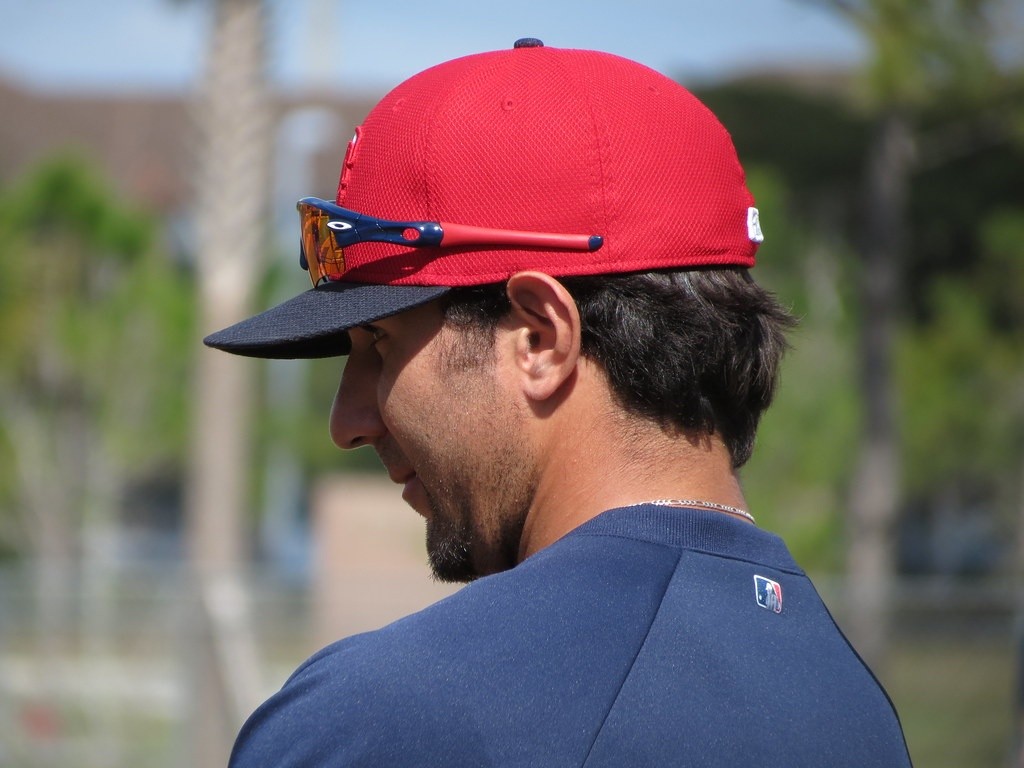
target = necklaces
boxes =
[629,501,758,522]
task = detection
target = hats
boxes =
[202,38,764,360]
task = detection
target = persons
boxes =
[203,50,915,768]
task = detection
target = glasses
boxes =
[295,195,604,291]
